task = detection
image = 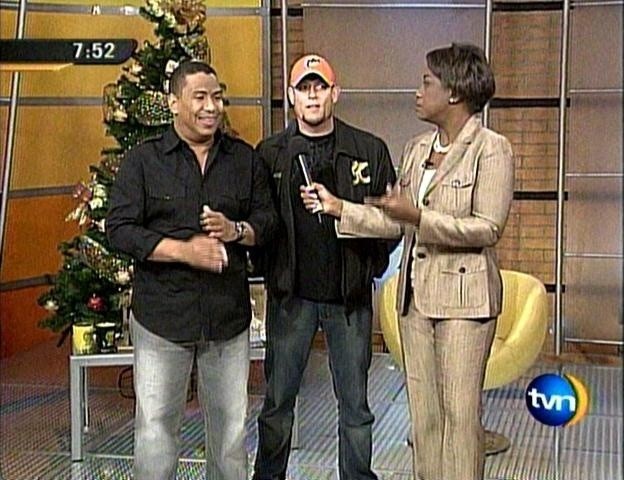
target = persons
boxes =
[249,52,403,480]
[298,43,516,480]
[104,59,283,479]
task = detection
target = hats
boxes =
[288,52,336,88]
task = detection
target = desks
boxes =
[70,344,298,464]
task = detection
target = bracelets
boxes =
[226,220,247,246]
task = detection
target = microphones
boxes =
[289,136,325,214]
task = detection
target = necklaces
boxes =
[433,132,450,155]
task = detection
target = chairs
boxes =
[380,267,548,456]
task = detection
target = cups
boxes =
[91,321,116,354]
[72,322,97,356]
